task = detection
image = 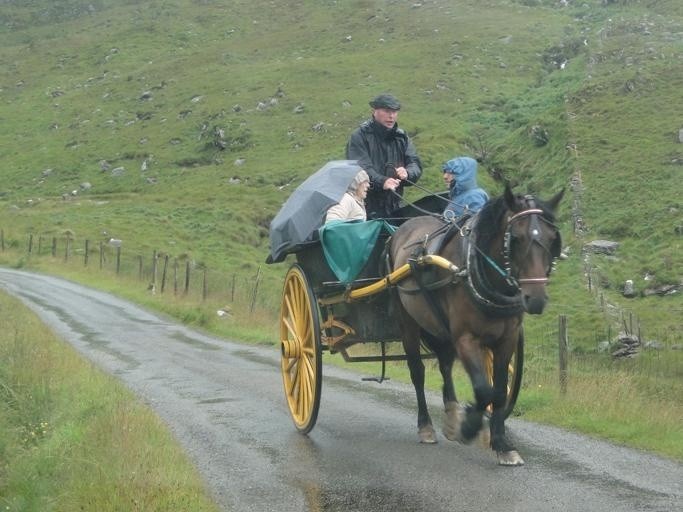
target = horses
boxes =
[375,178,567,468]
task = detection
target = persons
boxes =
[343,93,423,218]
[325,169,370,229]
[440,155,488,220]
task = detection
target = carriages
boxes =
[266,170,569,468]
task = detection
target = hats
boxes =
[370,94,401,110]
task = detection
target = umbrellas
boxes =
[267,160,361,263]
